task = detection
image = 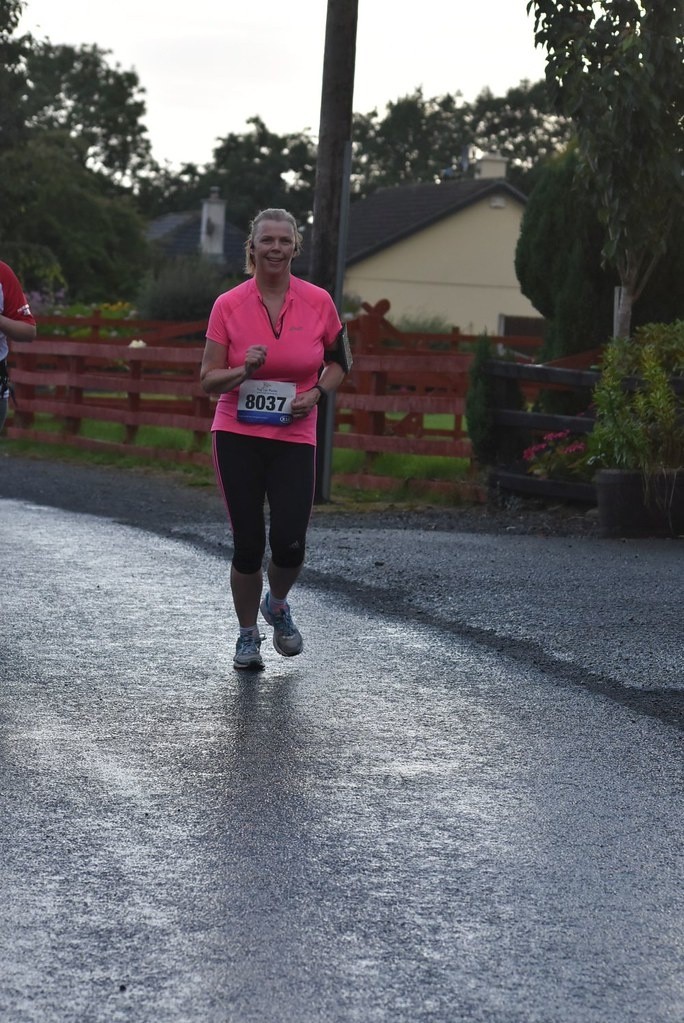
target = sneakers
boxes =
[260,591,304,657]
[233,629,267,668]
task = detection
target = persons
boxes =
[200,208,347,668]
[0,259,37,432]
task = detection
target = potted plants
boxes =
[591,319,684,533]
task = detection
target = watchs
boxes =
[312,385,328,405]
